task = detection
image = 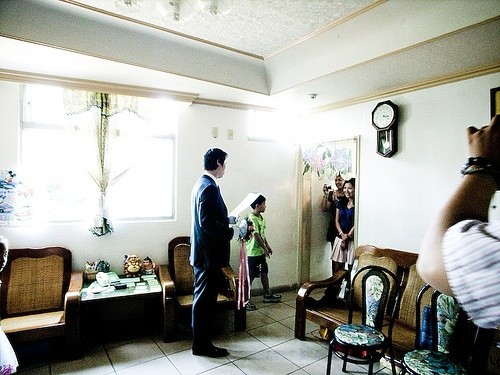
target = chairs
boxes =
[399,282,478,375]
[0,247,83,362]
[158,236,248,343]
[326,265,402,375]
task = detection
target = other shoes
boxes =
[245,299,256,311]
[192,343,228,357]
[262,293,282,303]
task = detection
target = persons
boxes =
[242,194,283,311]
[124,254,144,277]
[189,148,238,358]
[140,256,156,275]
[0,235,20,374]
[330,178,355,276]
[415,113,500,374]
[321,172,348,277]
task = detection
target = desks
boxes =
[82,271,162,347]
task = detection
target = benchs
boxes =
[295,245,436,361]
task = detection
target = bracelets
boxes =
[323,195,328,199]
[462,156,500,192]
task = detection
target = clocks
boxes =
[371,100,402,158]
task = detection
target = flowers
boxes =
[235,217,257,243]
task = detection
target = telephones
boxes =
[96,271,120,287]
